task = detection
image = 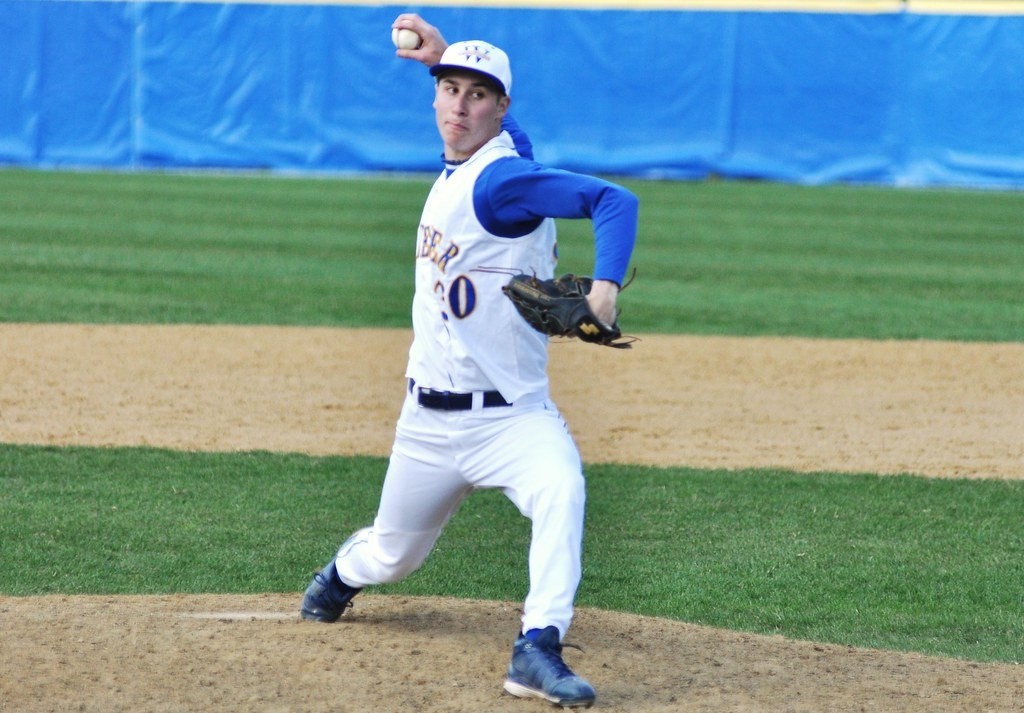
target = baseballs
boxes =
[392,25,421,51]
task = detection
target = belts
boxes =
[409,377,511,409]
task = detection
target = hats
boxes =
[430,40,512,97]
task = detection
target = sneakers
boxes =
[503,627,594,706]
[300,557,363,623]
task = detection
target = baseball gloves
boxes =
[502,273,621,346]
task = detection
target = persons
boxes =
[301,12,641,706]
